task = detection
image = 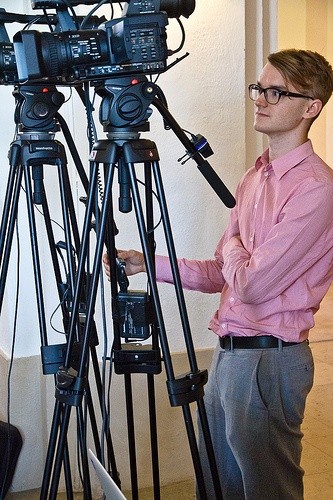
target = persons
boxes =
[101,46,333,500]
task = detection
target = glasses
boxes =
[248,83,315,106]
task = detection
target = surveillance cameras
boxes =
[0,1,197,86]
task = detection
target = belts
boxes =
[218,332,298,349]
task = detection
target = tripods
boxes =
[38,80,231,500]
[0,84,123,500]
